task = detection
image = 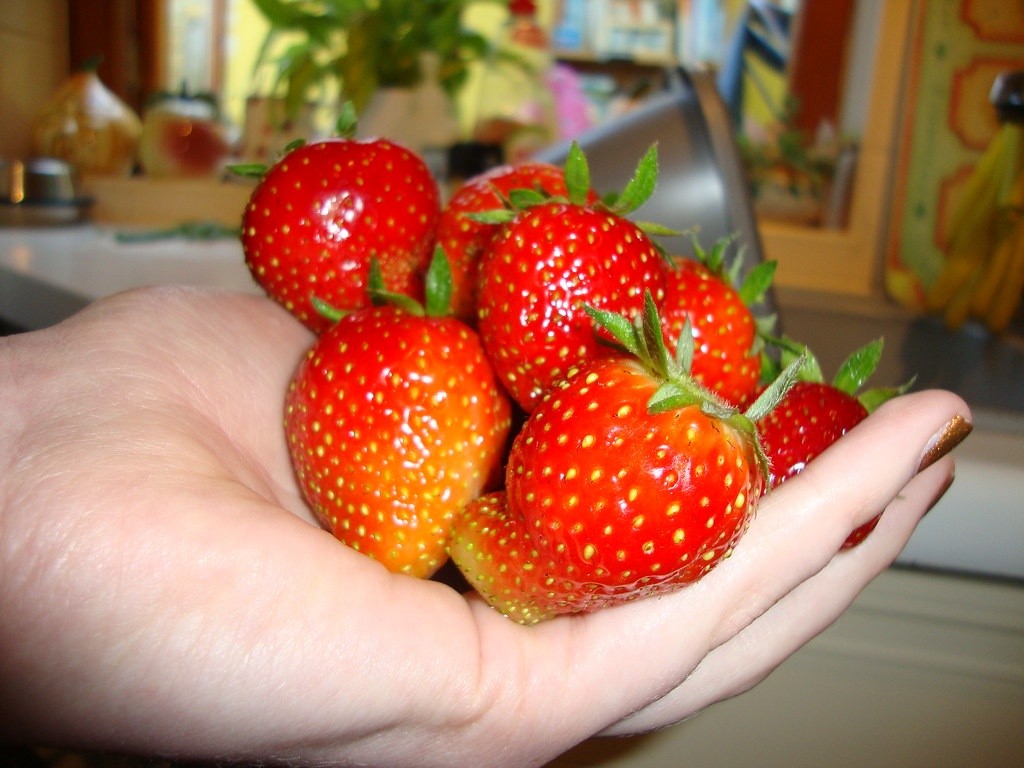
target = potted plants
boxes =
[250,0,558,156]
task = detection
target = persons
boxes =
[0,286,973,768]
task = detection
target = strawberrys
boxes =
[238,133,887,622]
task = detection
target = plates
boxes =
[2,195,92,219]
